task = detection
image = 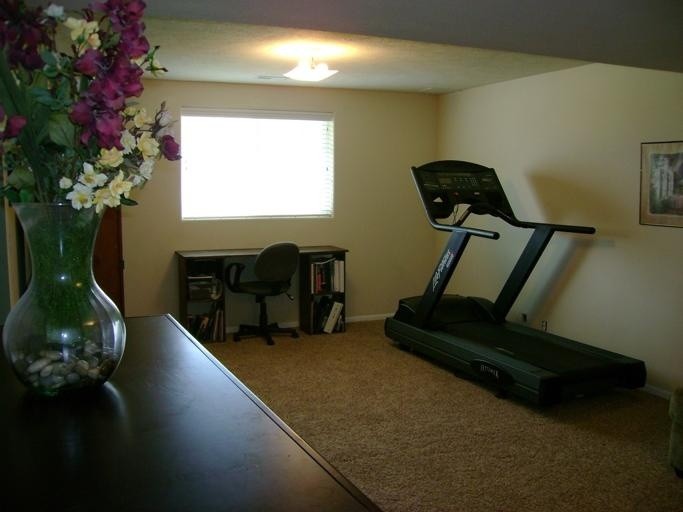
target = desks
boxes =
[1,311,384,512]
[175,244,349,344]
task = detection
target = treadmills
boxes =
[383,159,647,412]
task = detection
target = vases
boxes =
[2,202,129,397]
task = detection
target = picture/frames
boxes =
[638,141,682,228]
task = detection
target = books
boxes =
[187,271,212,281]
[309,254,345,335]
[186,302,223,342]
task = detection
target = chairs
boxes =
[225,242,300,345]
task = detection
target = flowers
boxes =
[1,0,184,351]
